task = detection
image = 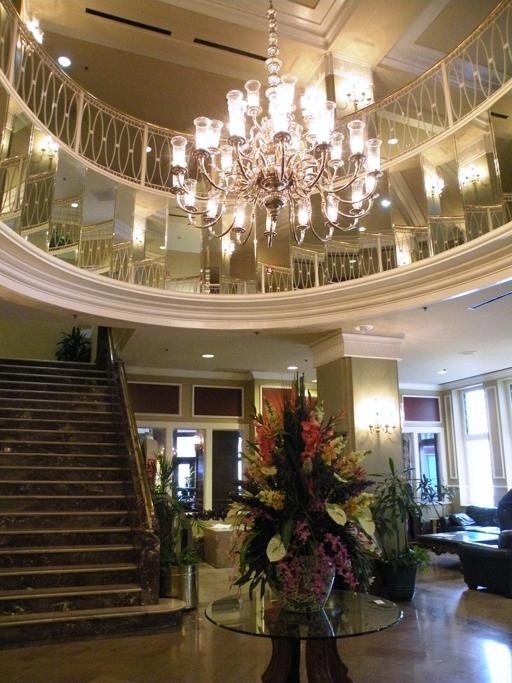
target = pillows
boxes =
[448,512,476,526]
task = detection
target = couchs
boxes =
[438,503,500,532]
[456,486,512,595]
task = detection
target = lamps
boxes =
[169,0,383,246]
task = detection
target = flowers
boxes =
[224,365,384,599]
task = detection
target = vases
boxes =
[272,552,339,613]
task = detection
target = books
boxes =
[212,522,232,529]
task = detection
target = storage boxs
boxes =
[201,525,245,569]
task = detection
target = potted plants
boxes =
[369,455,431,601]
[147,447,214,611]
[417,473,456,531]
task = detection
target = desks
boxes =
[428,516,449,533]
[203,587,405,681]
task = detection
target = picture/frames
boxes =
[191,382,245,420]
[259,385,293,415]
[127,380,184,417]
[402,394,443,423]
